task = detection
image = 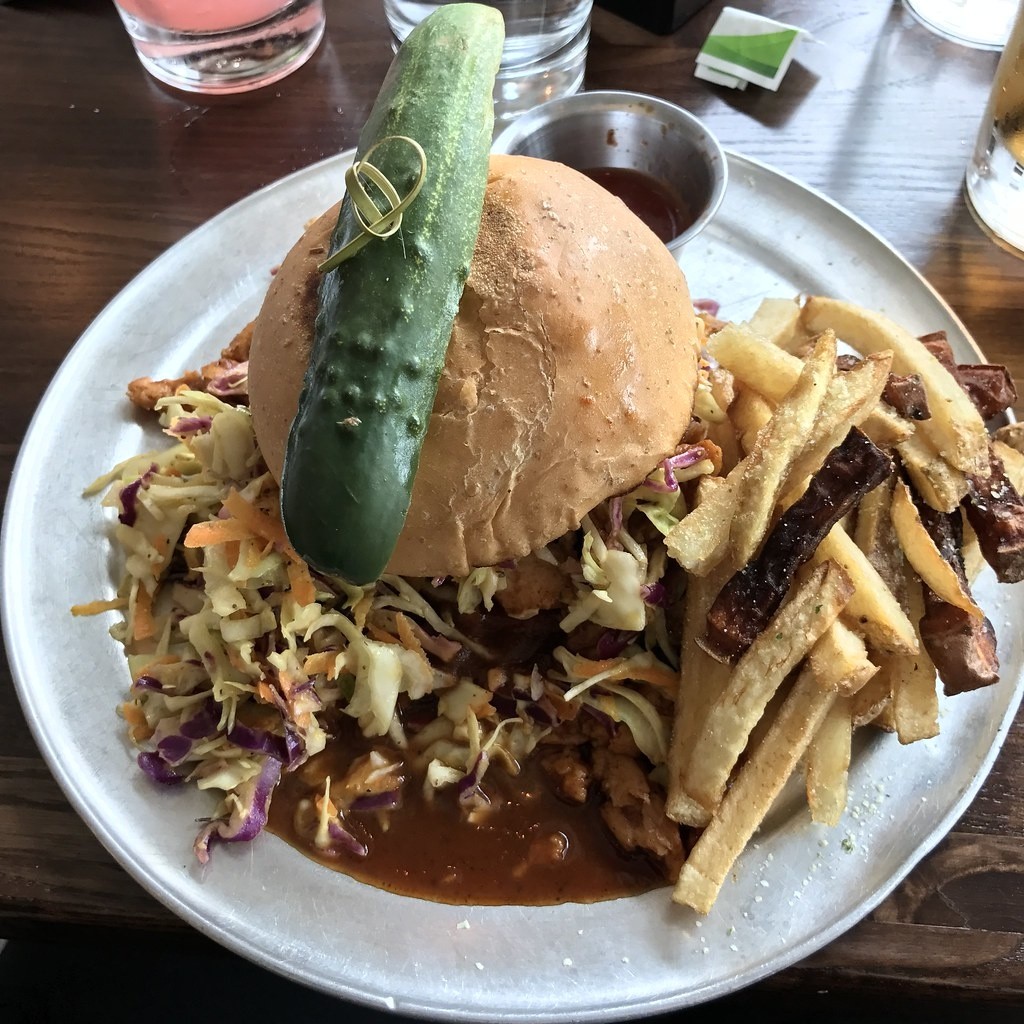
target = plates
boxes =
[0,145,1024,1024]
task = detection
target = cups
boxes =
[903,0,1019,52]
[492,89,728,252]
[382,0,592,117]
[965,0,1024,261]
[115,0,326,94]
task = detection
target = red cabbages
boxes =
[120,367,706,862]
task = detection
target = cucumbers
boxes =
[279,2,507,588]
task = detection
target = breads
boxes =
[243,154,724,579]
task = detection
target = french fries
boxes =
[660,293,1024,917]
